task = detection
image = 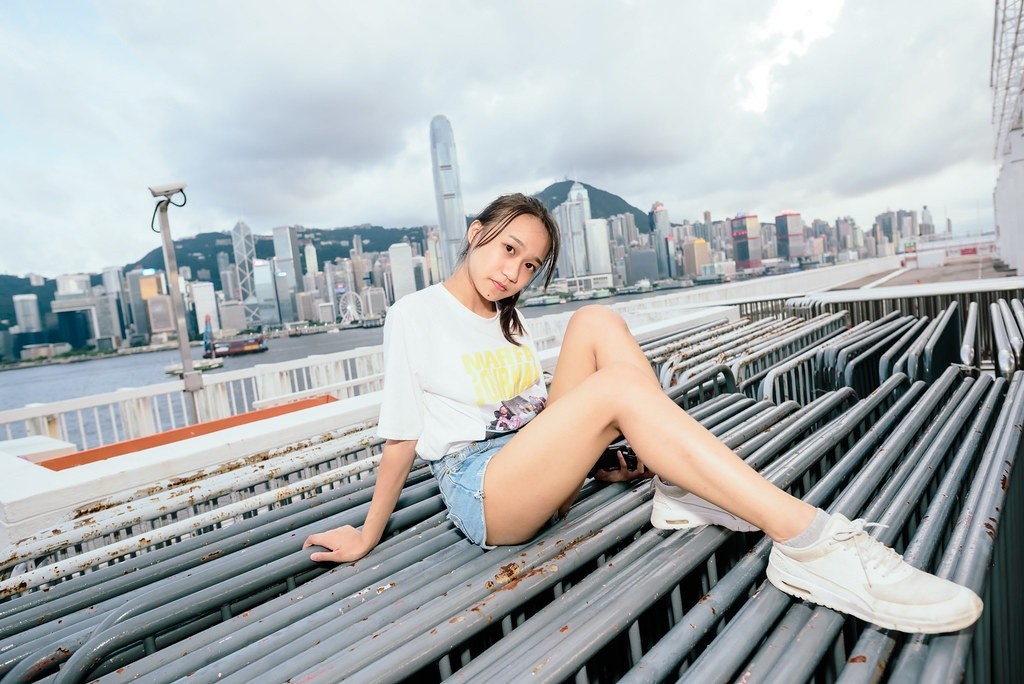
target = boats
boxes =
[524,295,561,305]
[616,278,653,295]
[361,317,383,328]
[573,288,610,300]
[652,278,695,291]
[168,358,226,375]
[203,334,269,358]
[326,328,339,334]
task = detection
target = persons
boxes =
[302,193,984,634]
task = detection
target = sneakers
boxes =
[650,475,761,532]
[766,512,983,634]
